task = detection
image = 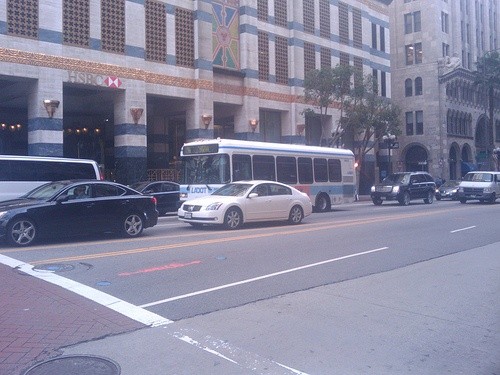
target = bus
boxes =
[180,137,358,213]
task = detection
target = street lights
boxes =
[382,134,396,175]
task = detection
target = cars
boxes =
[434,179,460,200]
[178,180,313,230]
[0,179,159,248]
[128,180,182,217]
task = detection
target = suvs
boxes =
[370,171,436,206]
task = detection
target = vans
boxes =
[457,171,500,204]
[0,154,104,204]
